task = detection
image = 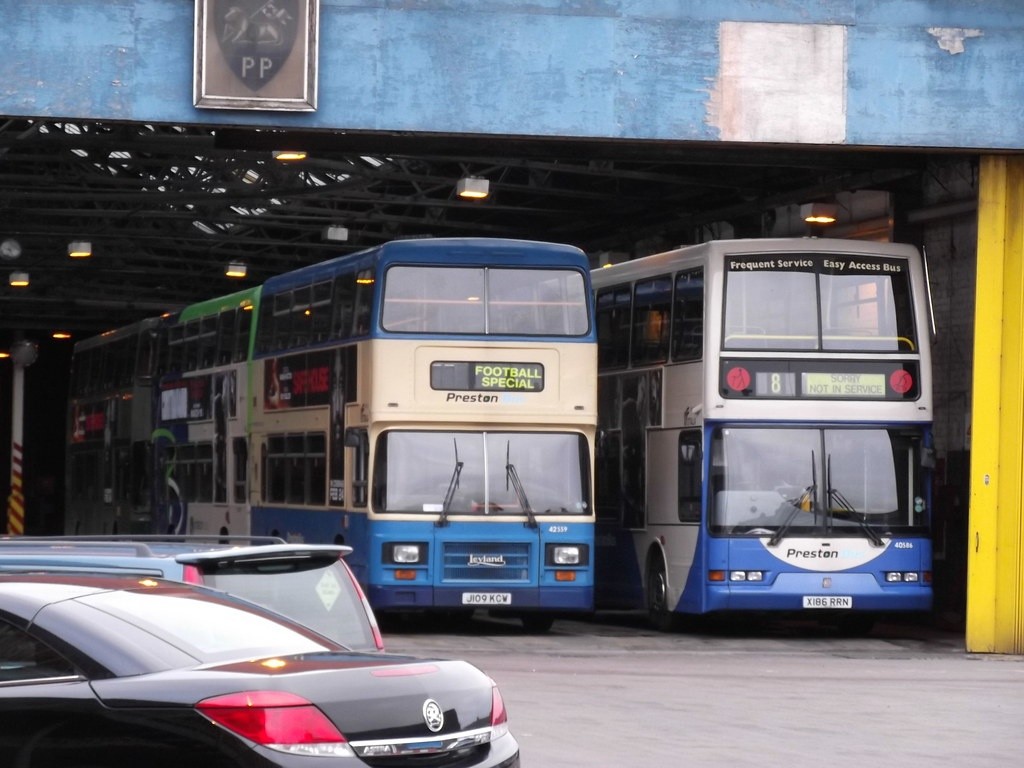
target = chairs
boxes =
[74,295,568,396]
[595,465,903,525]
[70,453,585,516]
[596,314,897,367]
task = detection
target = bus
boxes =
[62,308,173,537]
[156,236,601,631]
[589,235,948,631]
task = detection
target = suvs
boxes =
[0,529,387,657]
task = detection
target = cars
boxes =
[0,573,525,768]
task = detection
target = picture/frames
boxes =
[191,0,320,112]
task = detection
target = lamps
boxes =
[271,150,308,161]
[456,175,490,198]
[599,250,630,269]
[322,223,349,241]
[52,329,72,338]
[223,259,247,276]
[8,271,30,286]
[800,202,838,223]
[68,240,91,257]
